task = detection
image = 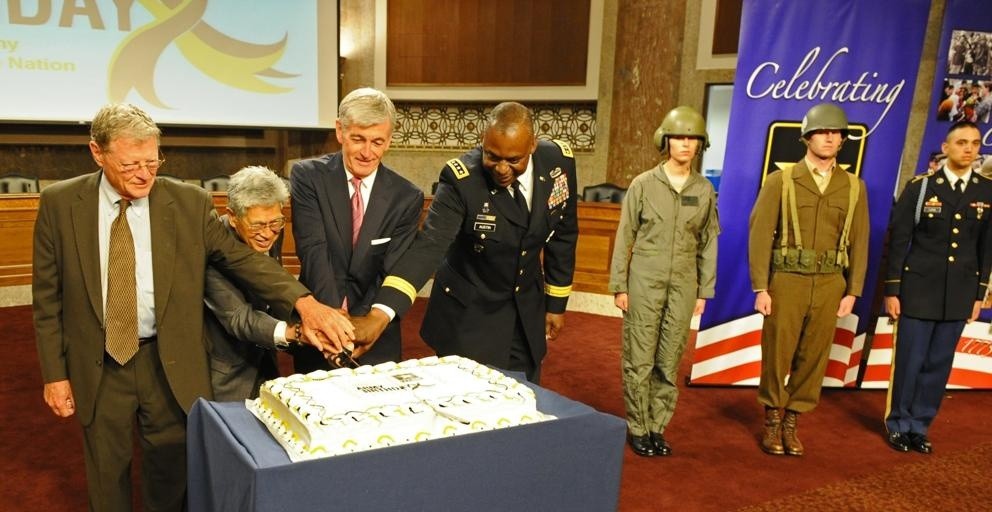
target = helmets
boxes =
[798,102,848,139]
[652,105,709,155]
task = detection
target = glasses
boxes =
[104,148,166,175]
[238,214,287,235]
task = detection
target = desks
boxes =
[186,363,630,510]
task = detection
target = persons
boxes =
[348,102,580,385]
[290,88,424,374]
[204,165,356,402]
[747,103,871,457]
[608,106,719,455]
[885,126,991,452]
[32,102,355,511]
[939,32,992,123]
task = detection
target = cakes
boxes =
[246,354,560,465]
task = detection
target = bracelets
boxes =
[295,323,303,347]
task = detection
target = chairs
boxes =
[201,175,230,192]
[1,174,40,193]
[581,184,628,204]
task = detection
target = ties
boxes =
[952,177,964,197]
[510,179,530,224]
[349,177,366,257]
[102,200,140,368]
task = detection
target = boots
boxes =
[781,407,804,458]
[761,402,785,457]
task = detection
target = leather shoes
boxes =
[886,427,911,453]
[907,429,933,455]
[645,430,673,458]
[624,428,656,458]
